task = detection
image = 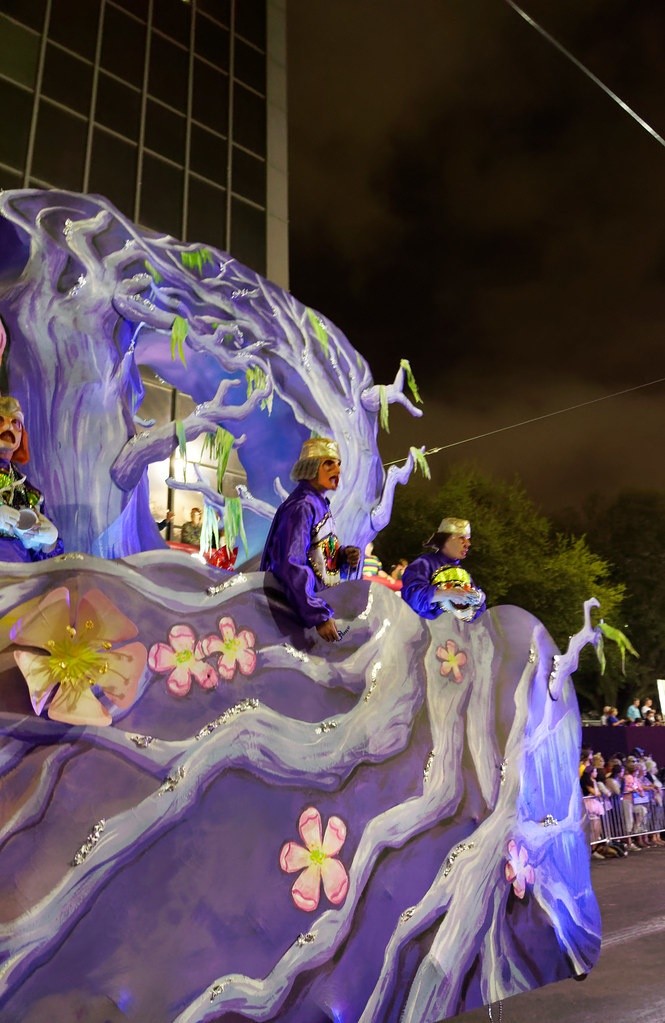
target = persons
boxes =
[401,517,487,623]
[0,397,65,562]
[580,746,665,859]
[392,559,408,579]
[601,697,663,726]
[259,438,361,643]
[157,511,175,531]
[181,508,202,544]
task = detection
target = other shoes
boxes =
[593,835,665,859]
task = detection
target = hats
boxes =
[425,517,472,547]
[298,439,343,460]
[0,397,25,426]
